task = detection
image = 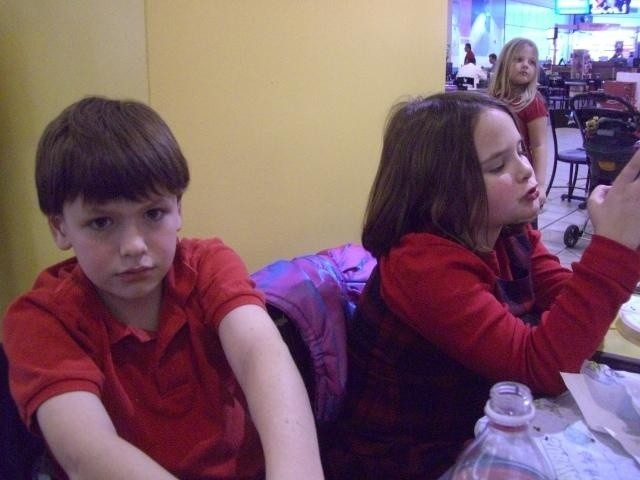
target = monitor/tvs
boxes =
[591,0,631,14]
[556,0,589,14]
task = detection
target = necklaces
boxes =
[480,53,497,73]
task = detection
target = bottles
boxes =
[447,379,560,480]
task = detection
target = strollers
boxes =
[563,91,640,249]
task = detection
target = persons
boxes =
[612,48,623,59]
[488,37,552,211]
[315,84,639,479]
[454,58,488,90]
[0,85,330,479]
[463,43,477,64]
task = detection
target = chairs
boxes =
[545,108,590,203]
[547,78,598,108]
[456,77,474,91]
